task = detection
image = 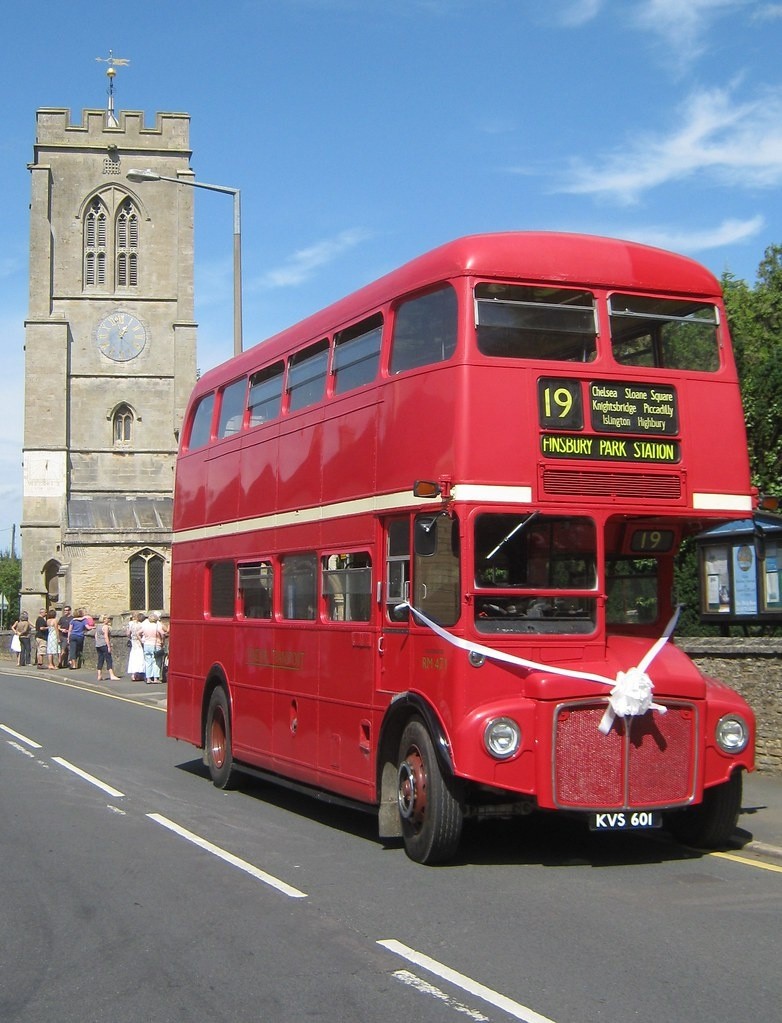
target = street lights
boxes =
[126,167,243,358]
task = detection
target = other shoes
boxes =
[16,663,20,666]
[146,680,162,683]
[48,665,59,670]
[37,665,48,669]
[132,676,145,681]
[69,667,78,670]
[57,665,67,669]
[97,677,103,681]
[27,663,33,667]
[110,675,120,681]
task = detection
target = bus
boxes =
[164,232,778,867]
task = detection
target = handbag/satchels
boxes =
[127,640,131,647]
[154,649,165,658]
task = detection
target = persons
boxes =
[46,610,61,670]
[127,613,146,681]
[136,614,166,684]
[16,614,33,667]
[71,607,93,627]
[57,606,73,668]
[126,611,140,629]
[66,609,91,669]
[11,611,35,666]
[35,608,48,669]
[144,611,169,633]
[95,614,121,681]
